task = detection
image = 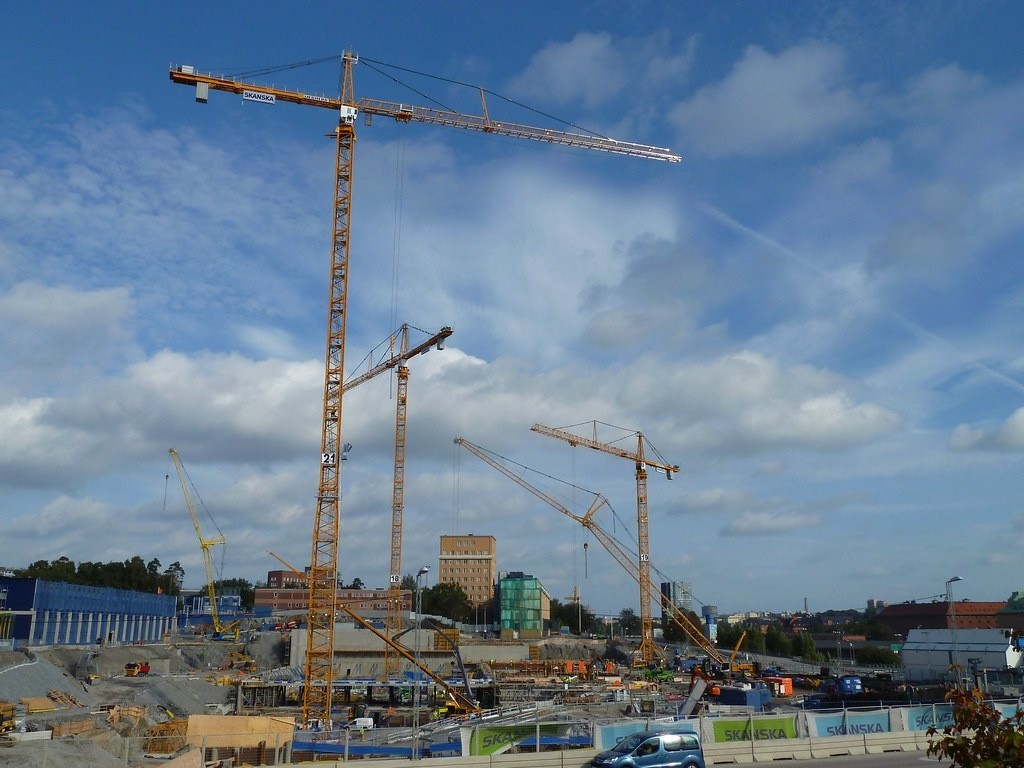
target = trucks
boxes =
[760,664,813,685]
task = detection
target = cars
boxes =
[591,729,706,768]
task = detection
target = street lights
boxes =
[943,572,970,741]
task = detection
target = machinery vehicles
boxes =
[940,661,988,709]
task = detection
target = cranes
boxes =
[164,43,750,745]
